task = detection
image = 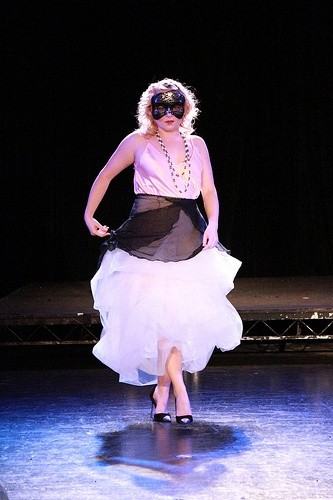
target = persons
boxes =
[84,78,242,422]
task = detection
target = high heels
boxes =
[149,384,171,422]
[175,397,193,424]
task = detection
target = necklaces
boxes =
[154,130,192,194]
